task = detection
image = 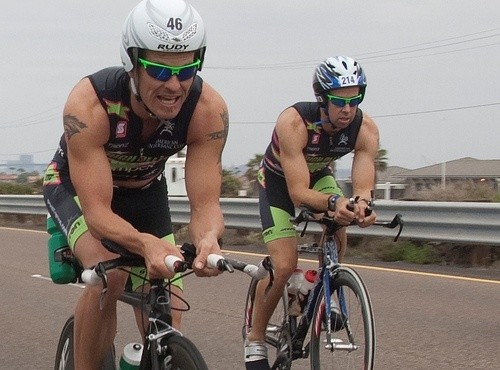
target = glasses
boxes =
[138,57,200,81]
[324,94,363,106]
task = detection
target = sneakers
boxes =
[322,298,343,331]
[244,332,269,370]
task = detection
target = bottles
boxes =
[288,268,304,316]
[46,212,76,284]
[119,343,143,370]
[298,270,317,303]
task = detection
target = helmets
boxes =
[120,0,207,73]
[312,55,367,107]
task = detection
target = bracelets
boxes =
[328,194,342,212]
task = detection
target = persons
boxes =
[241,55,380,369]
[40,0,229,369]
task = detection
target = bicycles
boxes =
[53,238,234,370]
[242,204,405,370]
